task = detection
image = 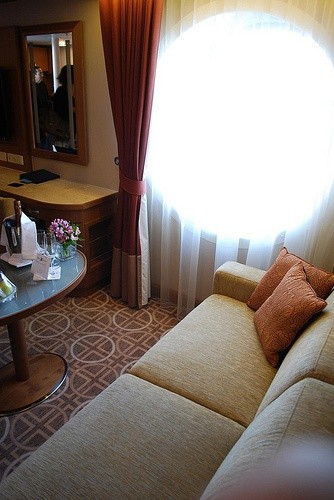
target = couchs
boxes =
[0,259,333,499]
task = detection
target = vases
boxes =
[50,238,77,262]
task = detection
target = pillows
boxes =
[246,246,334,313]
[252,261,328,369]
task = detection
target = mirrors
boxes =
[15,20,90,166]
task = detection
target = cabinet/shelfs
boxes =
[0,191,118,295]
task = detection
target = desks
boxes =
[0,236,88,417]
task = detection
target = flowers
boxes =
[46,217,85,257]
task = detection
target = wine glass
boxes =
[45,233,58,277]
[33,230,49,261]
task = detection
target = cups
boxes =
[2,218,21,254]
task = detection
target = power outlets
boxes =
[15,155,24,166]
[7,153,16,163]
[0,152,6,161]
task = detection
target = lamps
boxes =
[58,37,67,47]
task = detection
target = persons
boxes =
[32,64,78,145]
